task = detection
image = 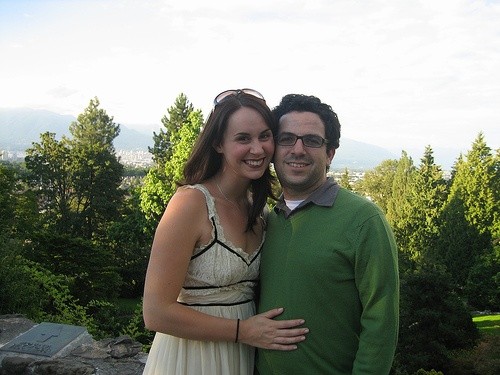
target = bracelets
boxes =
[235,318,240,343]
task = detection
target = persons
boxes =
[254,94,400,375]
[141,88,310,375]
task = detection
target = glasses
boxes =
[213,88,266,112]
[274,132,330,148]
